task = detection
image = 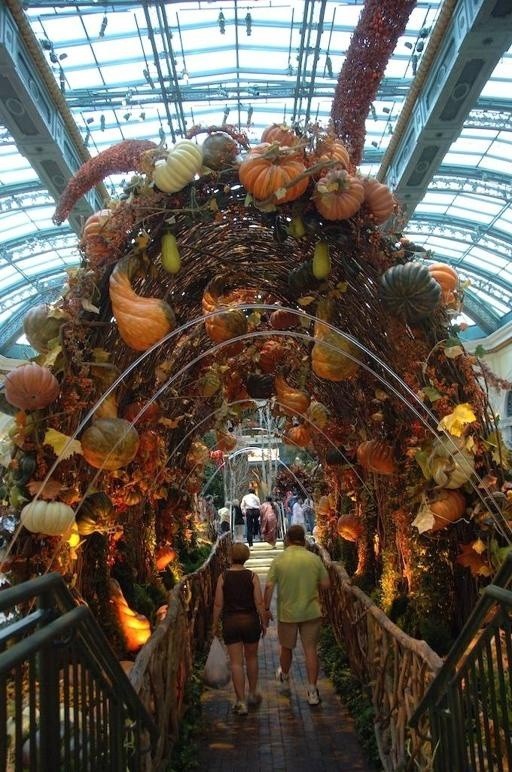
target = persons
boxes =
[262,524,330,705]
[206,486,315,548]
[211,542,267,715]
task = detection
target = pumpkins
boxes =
[3,255,465,570]
[151,123,397,222]
[76,208,120,266]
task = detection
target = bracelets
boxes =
[265,608,269,611]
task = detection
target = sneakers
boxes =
[275,666,291,692]
[231,701,249,716]
[305,688,321,705]
[248,688,264,708]
